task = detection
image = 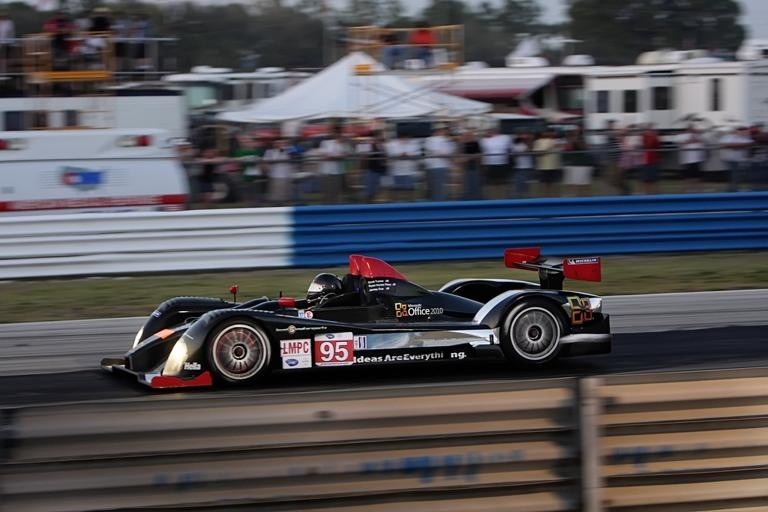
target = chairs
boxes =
[321,275,365,323]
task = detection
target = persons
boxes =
[408,21,437,64]
[1,5,150,94]
[378,22,403,70]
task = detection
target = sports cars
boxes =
[98,248,612,393]
